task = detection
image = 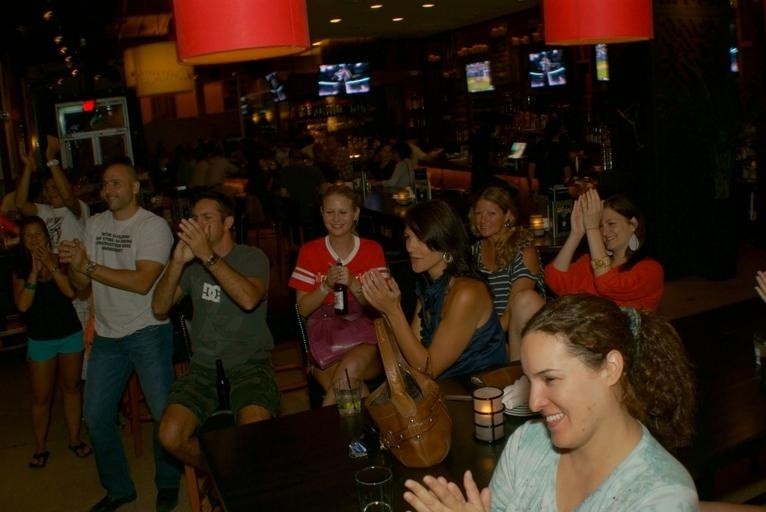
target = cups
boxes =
[754,331,766,366]
[332,378,362,417]
[354,465,394,512]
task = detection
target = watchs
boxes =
[204,253,224,269]
[82,262,99,274]
[50,266,61,275]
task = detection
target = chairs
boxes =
[293,303,324,410]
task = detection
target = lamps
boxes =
[471,389,507,446]
[392,187,413,206]
[122,48,138,88]
[529,213,545,237]
[172,0,312,65]
[543,0,654,46]
[132,41,196,97]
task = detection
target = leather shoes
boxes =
[156,489,179,512]
[89,489,136,511]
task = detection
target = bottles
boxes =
[455,121,471,141]
[335,260,350,316]
[504,91,538,114]
[296,98,368,119]
[402,91,426,127]
[734,144,762,180]
[214,359,229,411]
[512,106,557,129]
[579,121,617,171]
[441,95,452,120]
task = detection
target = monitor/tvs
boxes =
[528,49,567,88]
[594,43,610,81]
[318,62,370,97]
[465,60,495,93]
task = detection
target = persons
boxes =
[151,131,312,188]
[529,121,573,189]
[372,146,396,180]
[538,55,559,85]
[755,270,766,304]
[381,142,414,192]
[510,185,664,362]
[58,156,184,512]
[554,137,584,185]
[361,198,509,385]
[469,184,547,330]
[288,185,391,407]
[16,134,91,252]
[314,130,353,181]
[10,215,89,468]
[330,67,351,93]
[152,192,281,473]
[404,291,702,512]
[463,122,517,196]
[407,127,441,169]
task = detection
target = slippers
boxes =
[29,452,49,469]
[69,441,92,458]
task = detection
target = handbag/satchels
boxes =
[366,317,452,468]
[305,304,381,370]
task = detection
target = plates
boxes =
[502,401,543,417]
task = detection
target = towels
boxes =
[502,375,531,410]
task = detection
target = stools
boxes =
[184,410,236,512]
[128,331,190,458]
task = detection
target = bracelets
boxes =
[591,256,612,269]
[25,281,36,291]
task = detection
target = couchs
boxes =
[415,164,539,228]
